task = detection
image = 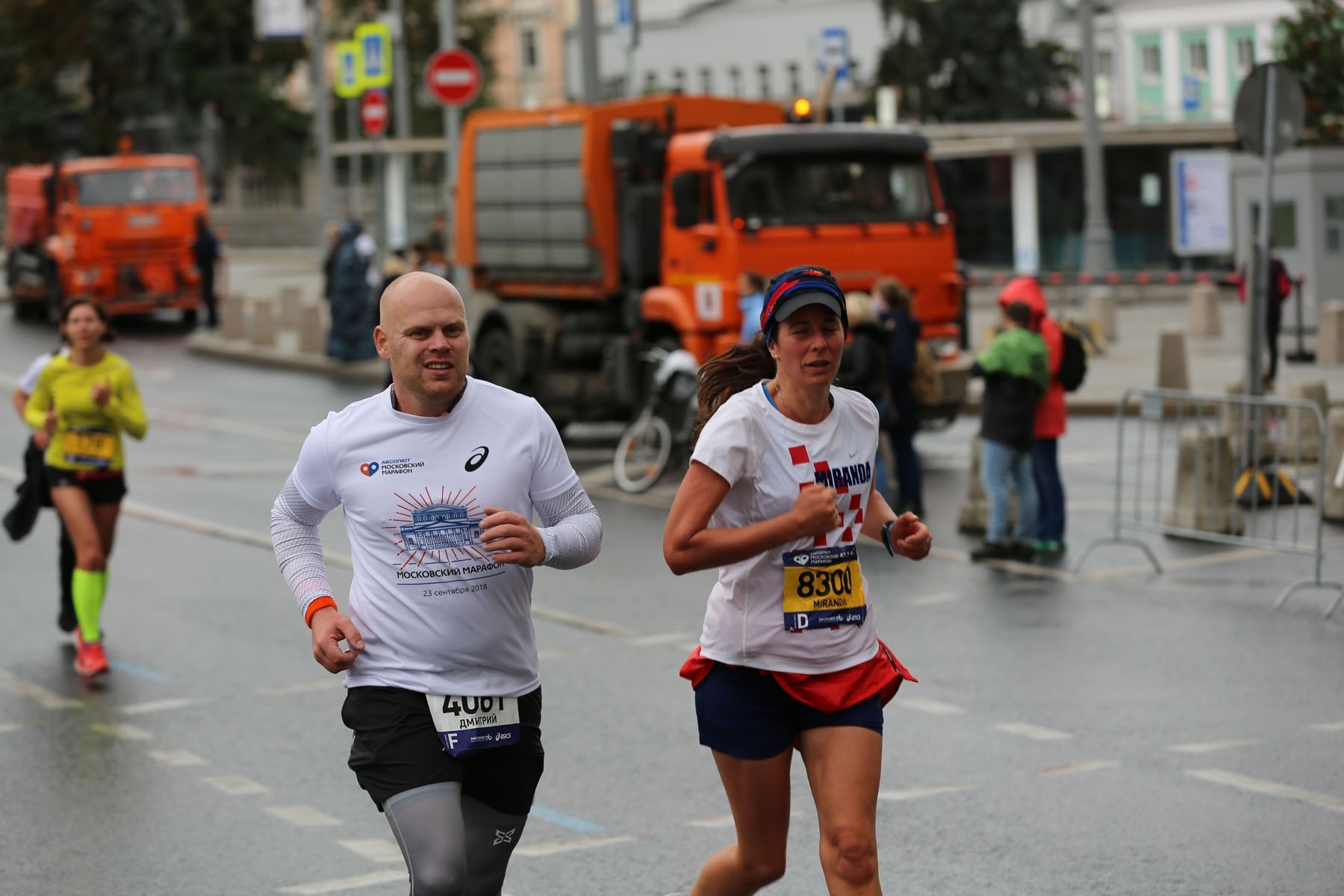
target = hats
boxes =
[773,290,842,322]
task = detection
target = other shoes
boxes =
[971,538,1035,564]
[59,596,77,631]
[1029,538,1066,567]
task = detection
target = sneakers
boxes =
[71,625,110,677]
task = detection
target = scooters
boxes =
[608,341,710,498]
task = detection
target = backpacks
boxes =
[1034,312,1086,391]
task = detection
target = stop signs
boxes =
[428,51,478,103]
[361,95,388,133]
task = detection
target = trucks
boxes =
[452,94,968,451]
[4,153,213,327]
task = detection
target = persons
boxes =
[966,302,1048,563]
[317,220,450,360]
[191,213,226,326]
[991,275,1069,557]
[3,297,145,679]
[1235,236,1296,387]
[839,281,932,524]
[271,273,603,896]
[660,264,932,896]
[736,272,766,343]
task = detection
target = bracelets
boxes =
[879,520,897,558]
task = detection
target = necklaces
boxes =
[772,382,832,422]
